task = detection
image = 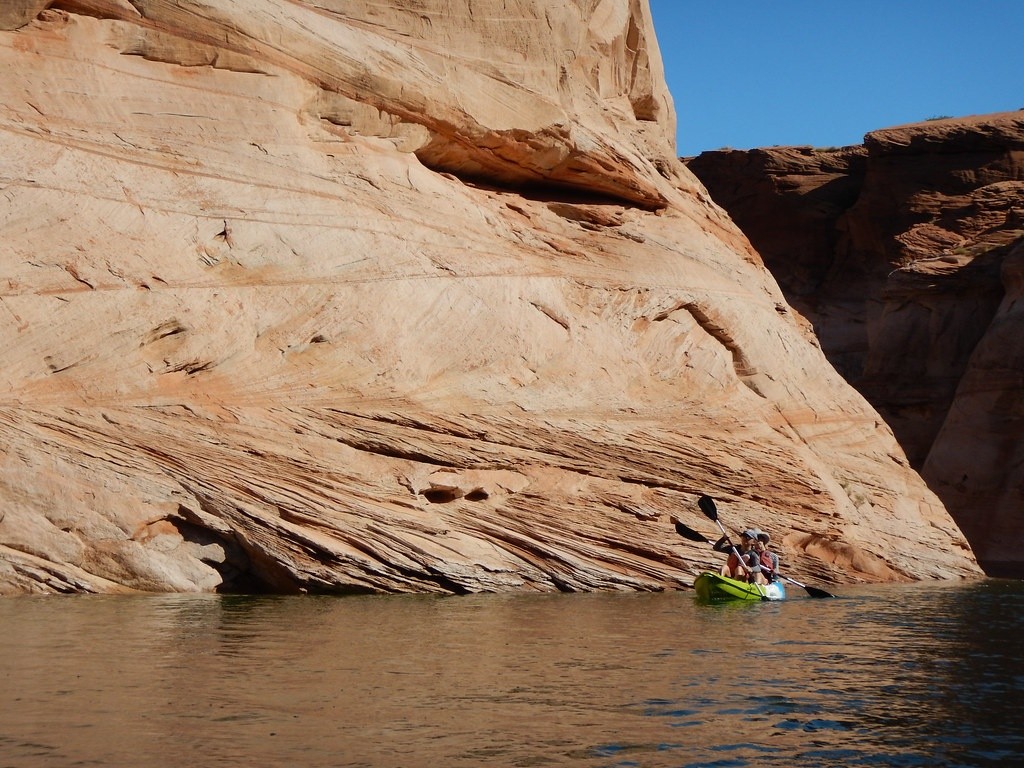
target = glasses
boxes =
[743,534,751,539]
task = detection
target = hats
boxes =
[741,528,755,538]
[754,527,770,544]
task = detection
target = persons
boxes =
[713,528,761,584]
[752,526,779,584]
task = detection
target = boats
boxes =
[692,569,784,607]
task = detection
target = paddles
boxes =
[697,495,772,601]
[674,520,836,599]
